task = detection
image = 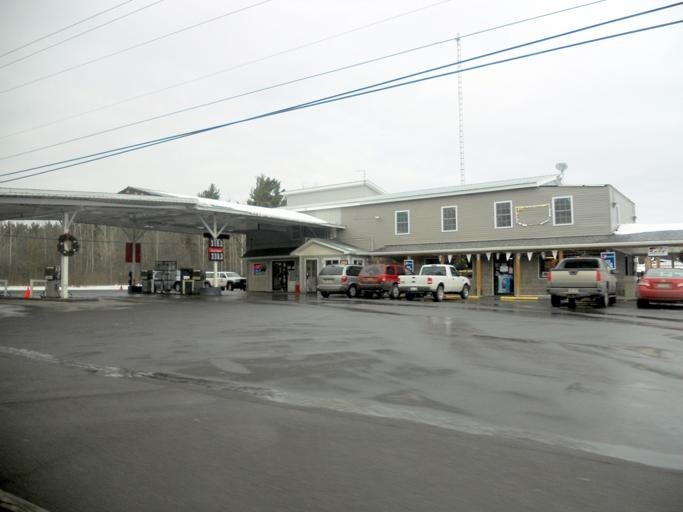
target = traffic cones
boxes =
[24,287,31,298]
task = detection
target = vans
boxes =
[316,261,363,297]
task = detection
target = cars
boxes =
[634,267,682,307]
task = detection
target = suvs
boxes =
[356,263,405,300]
[150,264,246,292]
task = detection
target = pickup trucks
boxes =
[545,255,618,310]
[398,262,471,301]
